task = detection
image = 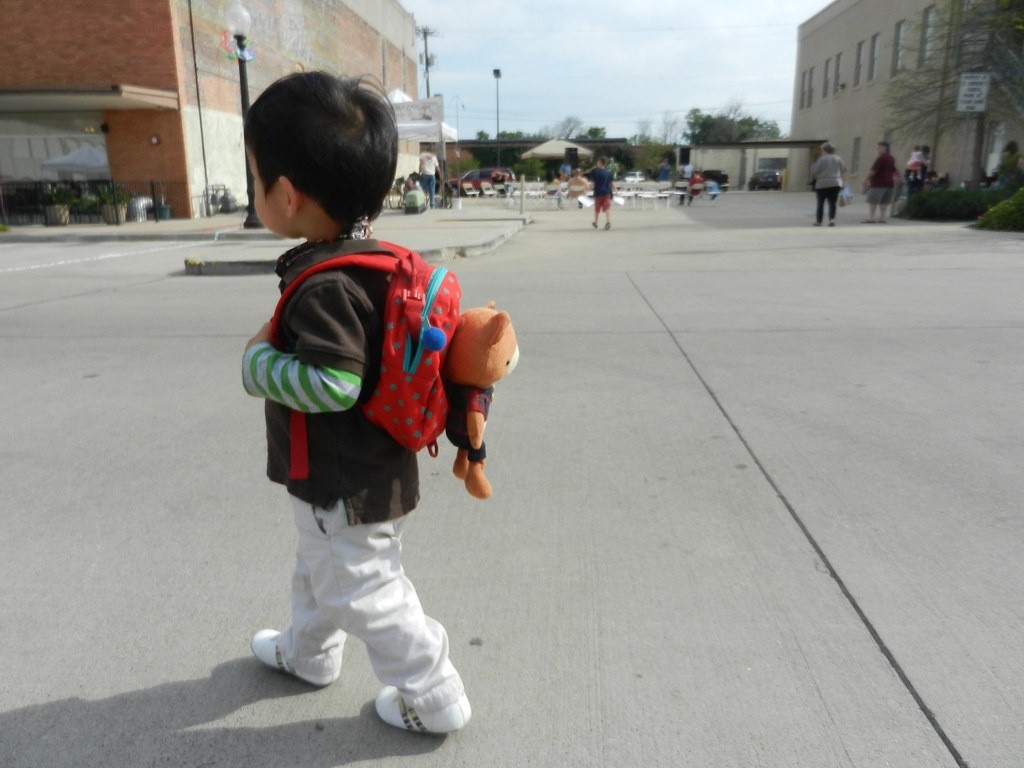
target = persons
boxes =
[658,158,671,199]
[904,145,945,194]
[405,146,443,210]
[680,164,719,205]
[544,157,618,229]
[863,142,896,222]
[243,71,470,734]
[491,172,512,195]
[965,141,1024,193]
[811,143,844,226]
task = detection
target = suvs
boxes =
[449,167,516,192]
[701,169,729,192]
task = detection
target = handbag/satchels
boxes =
[839,187,853,206]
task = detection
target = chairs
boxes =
[462,181,730,212]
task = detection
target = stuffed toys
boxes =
[446,301,519,499]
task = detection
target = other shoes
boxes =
[812,223,820,226]
[917,175,921,180]
[909,176,912,182]
[593,222,597,228]
[829,222,834,227]
[878,220,886,223]
[377,686,471,733]
[249,630,340,686]
[604,223,610,230]
[862,219,874,223]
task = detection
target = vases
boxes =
[157,203,171,219]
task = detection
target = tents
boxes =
[40,147,109,179]
[383,90,461,208]
[521,139,593,161]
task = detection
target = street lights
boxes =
[415,24,436,98]
[227,0,263,228]
[455,96,464,198]
[493,69,500,166]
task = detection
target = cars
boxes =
[748,170,782,190]
[624,172,645,182]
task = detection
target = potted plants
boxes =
[43,188,80,225]
[97,180,128,225]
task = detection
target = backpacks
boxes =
[267,241,461,457]
